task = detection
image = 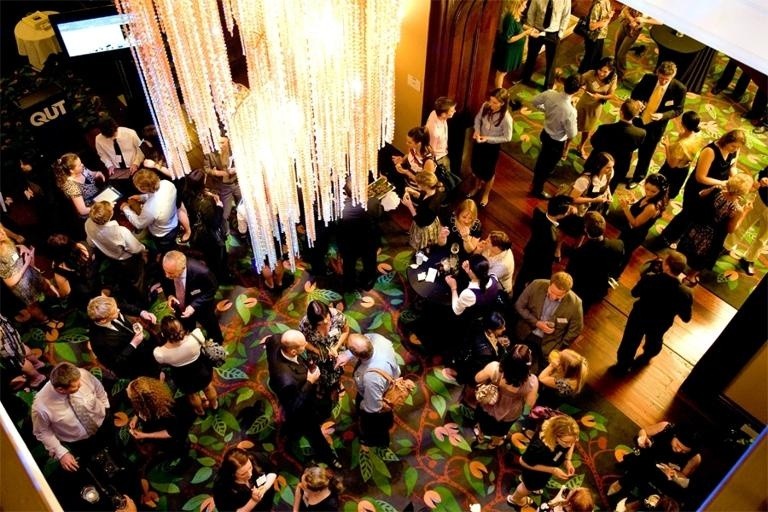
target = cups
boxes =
[545,319,555,330]
[133,323,143,334]
[80,486,100,503]
[748,193,756,203]
[451,243,459,254]
[644,436,654,448]
[441,255,458,271]
[502,335,508,345]
[548,349,560,363]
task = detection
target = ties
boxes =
[641,85,666,125]
[68,395,99,437]
[111,320,134,337]
[175,277,185,310]
[543,2,553,29]
[113,139,126,169]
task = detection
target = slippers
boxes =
[507,495,533,507]
[518,475,544,495]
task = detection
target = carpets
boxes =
[1,241,679,512]
[500,16,768,311]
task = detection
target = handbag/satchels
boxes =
[383,376,414,411]
[320,357,343,389]
[434,164,461,193]
[201,338,226,367]
[557,182,591,219]
[475,384,499,405]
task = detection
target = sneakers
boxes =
[739,257,755,275]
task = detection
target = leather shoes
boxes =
[626,180,638,189]
[529,191,550,199]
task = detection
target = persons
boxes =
[0,1,767,512]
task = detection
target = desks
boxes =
[14,10,61,69]
[300,197,379,290]
[407,245,473,300]
[650,24,706,62]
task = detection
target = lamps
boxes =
[111,0,399,274]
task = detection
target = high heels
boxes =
[480,192,489,207]
[608,480,621,495]
[466,187,481,198]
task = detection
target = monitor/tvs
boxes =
[48,2,153,64]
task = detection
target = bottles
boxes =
[309,360,320,380]
